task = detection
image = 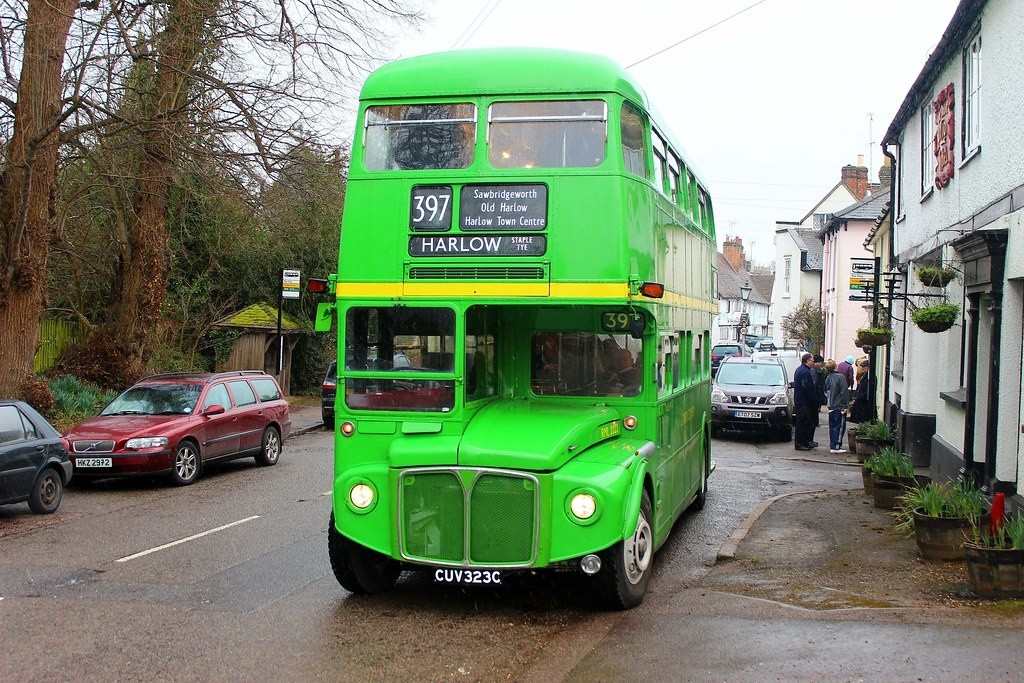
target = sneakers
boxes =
[830,449,847,454]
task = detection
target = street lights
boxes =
[740,279,753,356]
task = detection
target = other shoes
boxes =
[818,410,820,413]
[796,441,818,451]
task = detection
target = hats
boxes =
[814,355,824,362]
[860,360,868,367]
[844,355,855,365]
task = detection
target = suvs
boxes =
[62,369,290,486]
[711,354,796,442]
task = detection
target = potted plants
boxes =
[855,419,896,463]
[847,421,874,453]
[861,446,903,496]
[916,263,958,287]
[961,508,1024,598]
[858,329,896,346]
[862,344,872,354]
[908,480,991,564]
[871,453,932,510]
[912,299,960,333]
[852,337,864,348]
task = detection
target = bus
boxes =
[308,47,721,613]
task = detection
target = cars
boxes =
[711,332,808,390]
[0,400,73,515]
[321,350,415,431]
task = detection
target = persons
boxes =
[794,352,877,455]
[593,349,695,395]
